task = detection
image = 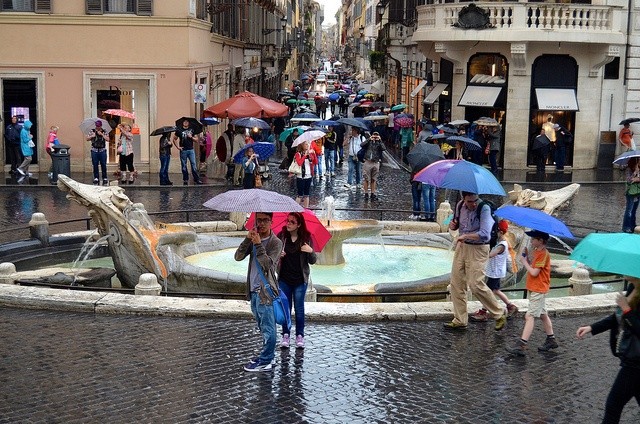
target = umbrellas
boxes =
[413,159,508,197]
[201,117,221,125]
[230,117,271,129]
[571,232,640,278]
[619,118,639,124]
[279,127,303,142]
[203,89,289,118]
[290,113,322,121]
[365,115,388,120]
[472,117,500,127]
[494,205,576,240]
[206,133,212,159]
[406,142,446,172]
[391,104,407,111]
[337,117,368,129]
[439,124,458,135]
[278,63,391,114]
[395,117,414,128]
[149,125,177,136]
[426,134,447,141]
[176,117,203,134]
[233,142,275,164]
[78,117,112,136]
[315,120,340,126]
[425,135,449,141]
[292,130,326,148]
[243,209,331,252]
[307,127,330,132]
[450,120,470,125]
[612,151,640,166]
[201,188,303,213]
[102,109,136,120]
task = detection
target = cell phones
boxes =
[308,150,313,152]
[254,153,259,158]
[255,224,259,231]
[373,135,378,139]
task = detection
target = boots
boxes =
[121,172,126,181]
[129,173,135,183]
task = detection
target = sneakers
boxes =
[472,310,488,320]
[251,357,276,364]
[296,336,305,349]
[410,215,417,219]
[506,343,526,355]
[344,183,351,188]
[444,322,466,330]
[416,215,421,219]
[364,194,369,200]
[325,172,329,176]
[356,184,361,188]
[280,335,290,346]
[538,340,558,351]
[371,194,377,200]
[93,178,99,182]
[505,304,518,320]
[244,362,272,371]
[17,168,24,175]
[25,173,32,176]
[495,308,507,331]
[104,178,108,181]
[331,172,336,176]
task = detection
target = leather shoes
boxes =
[160,182,167,185]
[196,181,203,184]
[166,181,173,184]
[429,217,434,220]
[184,181,187,184]
[420,217,429,221]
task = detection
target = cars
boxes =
[317,74,325,83]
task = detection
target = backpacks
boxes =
[90,129,106,148]
[458,199,498,246]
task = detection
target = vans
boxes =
[327,73,338,82]
[325,80,335,91]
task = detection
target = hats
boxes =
[498,219,508,232]
[525,230,549,240]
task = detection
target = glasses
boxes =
[256,219,272,224]
[285,220,297,226]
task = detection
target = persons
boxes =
[5,115,23,176]
[618,123,635,153]
[225,125,236,180]
[310,138,322,179]
[542,115,560,143]
[294,141,318,209]
[86,120,109,184]
[532,129,553,177]
[505,230,559,356]
[409,170,436,220]
[623,158,640,232]
[576,276,640,424]
[473,221,519,321]
[324,126,337,176]
[446,142,469,158]
[173,120,203,184]
[199,129,205,164]
[447,136,481,150]
[276,213,316,348]
[446,189,512,331]
[16,121,35,176]
[285,129,299,177]
[553,123,574,172]
[343,127,366,190]
[271,116,285,151]
[387,110,394,138]
[232,129,246,185]
[159,132,173,185]
[370,120,388,142]
[235,213,283,372]
[46,126,59,178]
[114,124,140,185]
[328,114,346,164]
[360,132,386,199]
[241,147,256,188]
[415,124,434,144]
[471,126,486,167]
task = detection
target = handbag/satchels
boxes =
[49,134,59,152]
[289,153,305,174]
[275,235,287,275]
[29,140,35,147]
[252,241,291,331]
[357,141,368,163]
[506,244,518,274]
[116,135,123,155]
[254,162,262,187]
[625,177,640,196]
